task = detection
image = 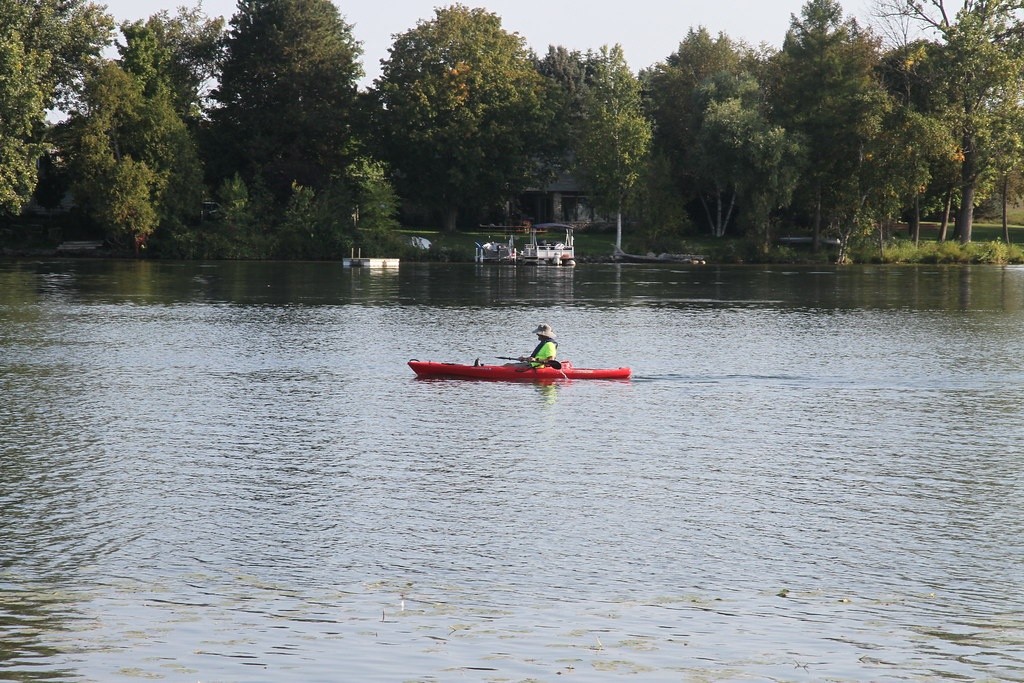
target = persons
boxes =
[474,323,558,368]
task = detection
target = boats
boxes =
[406,357,632,383]
[521,222,576,266]
[611,244,705,265]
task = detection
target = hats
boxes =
[532,323,556,338]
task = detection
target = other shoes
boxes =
[475,358,481,366]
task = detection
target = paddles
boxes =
[495,355,562,370]
